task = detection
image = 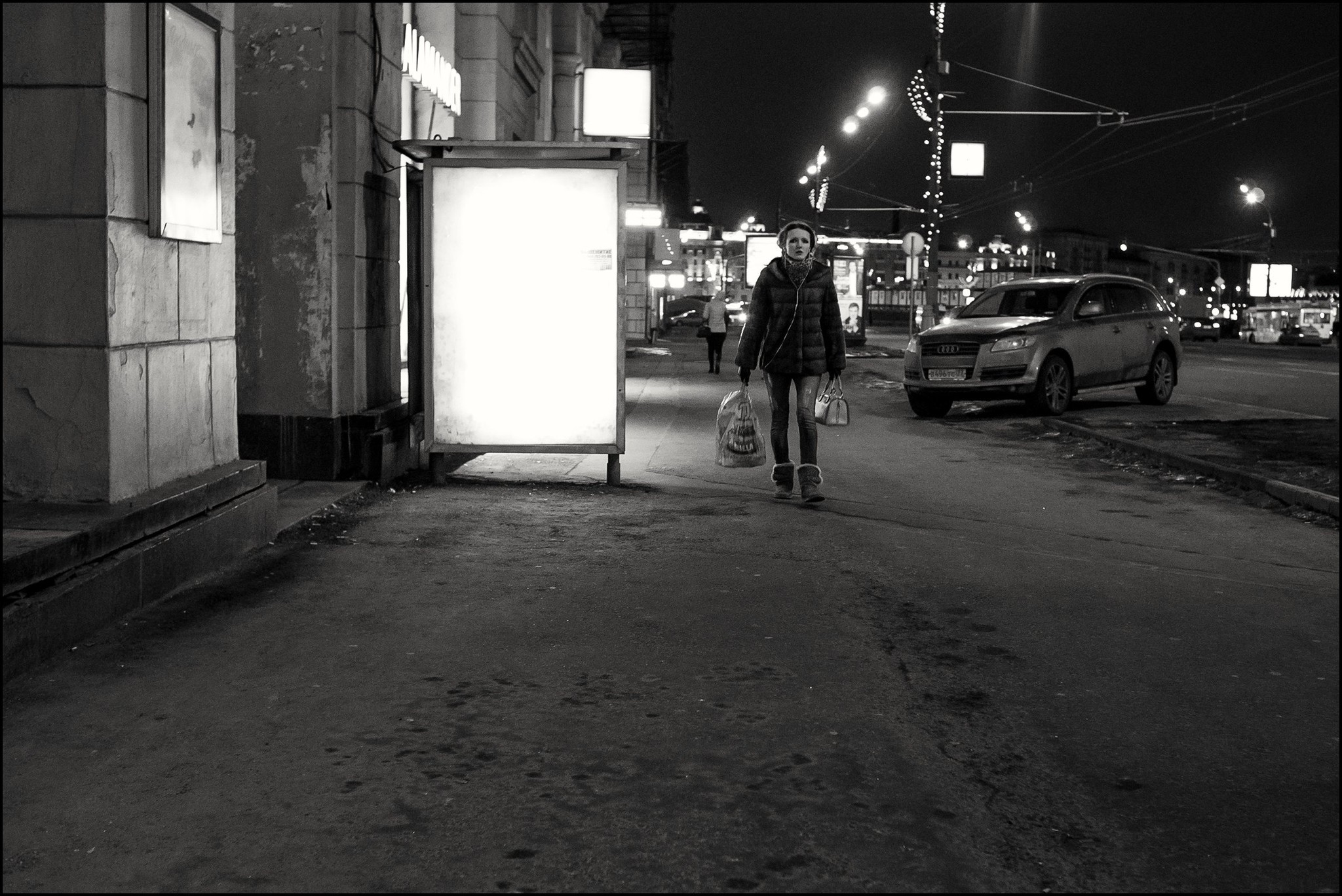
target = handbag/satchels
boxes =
[814,375,849,426]
[696,319,711,338]
[716,382,767,468]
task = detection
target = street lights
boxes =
[1238,182,1277,239]
[1120,241,1222,314]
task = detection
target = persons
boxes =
[834,261,863,297]
[734,221,846,503]
[842,302,862,338]
[702,291,730,374]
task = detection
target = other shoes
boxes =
[715,365,720,375]
[708,369,714,374]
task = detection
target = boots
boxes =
[771,460,795,499]
[796,463,825,502]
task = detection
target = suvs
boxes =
[904,272,1183,416]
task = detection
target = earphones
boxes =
[809,247,811,252]
[783,247,786,252]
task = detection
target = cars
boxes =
[726,307,746,325]
[1182,317,1221,342]
[1279,325,1322,347]
[668,310,709,327]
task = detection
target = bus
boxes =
[1239,305,1334,343]
